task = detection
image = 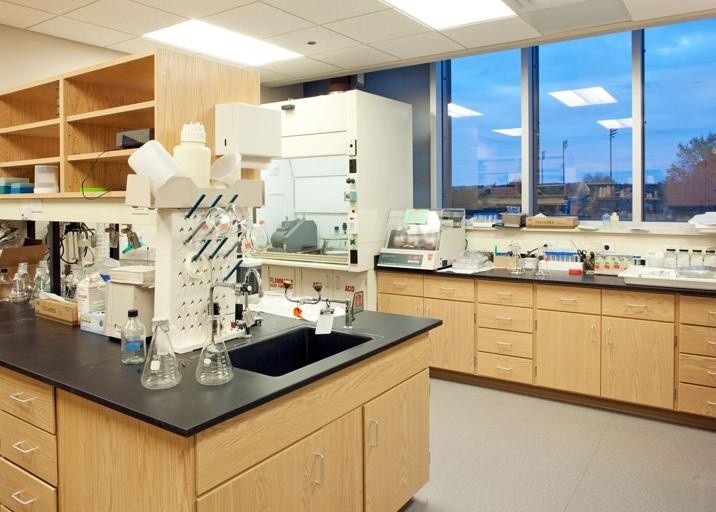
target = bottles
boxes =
[119,309,147,366]
[11,260,51,309]
[139,317,182,391]
[0,267,13,302]
[184,202,269,256]
[602,211,619,231]
[661,248,715,271]
[507,243,526,275]
[532,247,551,279]
[171,121,212,187]
[194,315,235,387]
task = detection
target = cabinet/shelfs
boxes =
[195,368,429,512]
[57,390,194,511]
[301,269,367,308]
[0,367,56,512]
[0,49,261,198]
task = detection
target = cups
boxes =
[127,139,185,193]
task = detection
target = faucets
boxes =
[244,268,264,311]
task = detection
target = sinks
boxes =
[211,322,384,379]
